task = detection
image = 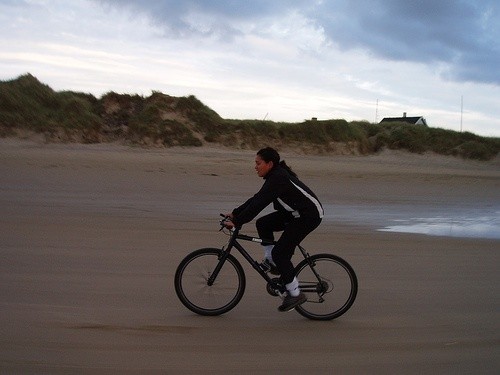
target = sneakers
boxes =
[260,258,280,275]
[277,290,307,313]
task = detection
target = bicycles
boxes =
[173,214,358,322]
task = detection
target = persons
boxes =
[224,147,324,312]
